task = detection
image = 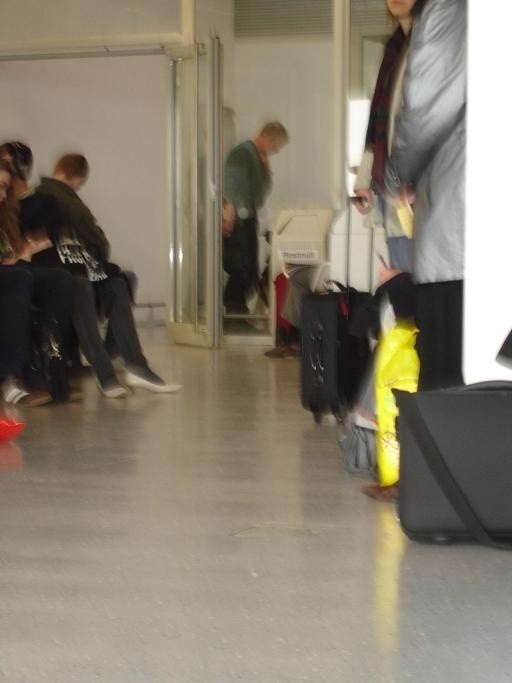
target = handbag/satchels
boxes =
[343,353,377,485]
[33,319,69,401]
[391,381,512,542]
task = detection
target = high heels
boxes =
[95,377,128,398]
[125,372,182,394]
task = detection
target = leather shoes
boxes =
[360,481,399,504]
[265,341,301,357]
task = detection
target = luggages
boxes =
[300,196,373,424]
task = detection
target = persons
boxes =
[1,141,184,407]
[362,0,467,501]
[379,268,415,322]
[222,122,290,333]
[348,0,428,270]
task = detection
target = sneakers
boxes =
[67,377,85,400]
[1,384,52,408]
